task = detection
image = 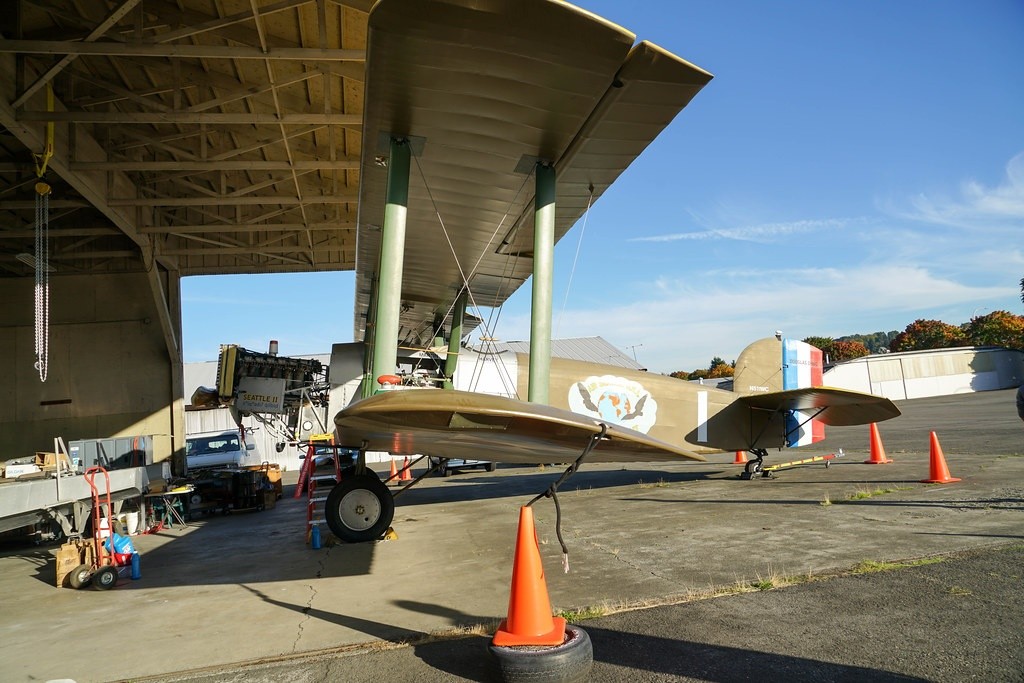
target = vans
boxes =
[187,428,261,467]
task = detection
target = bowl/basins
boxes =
[110,553,131,567]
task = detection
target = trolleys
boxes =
[69,466,119,591]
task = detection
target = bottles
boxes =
[131,550,141,578]
[311,524,320,548]
[105,533,134,553]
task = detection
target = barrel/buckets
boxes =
[126,512,139,535]
[94,518,109,538]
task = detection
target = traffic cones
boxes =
[921,431,962,485]
[388,458,399,480]
[492,506,566,646]
[400,458,413,481]
[864,423,894,465]
[732,451,750,464]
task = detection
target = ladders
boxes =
[307,434,343,543]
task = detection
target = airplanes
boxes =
[189,0,903,545]
